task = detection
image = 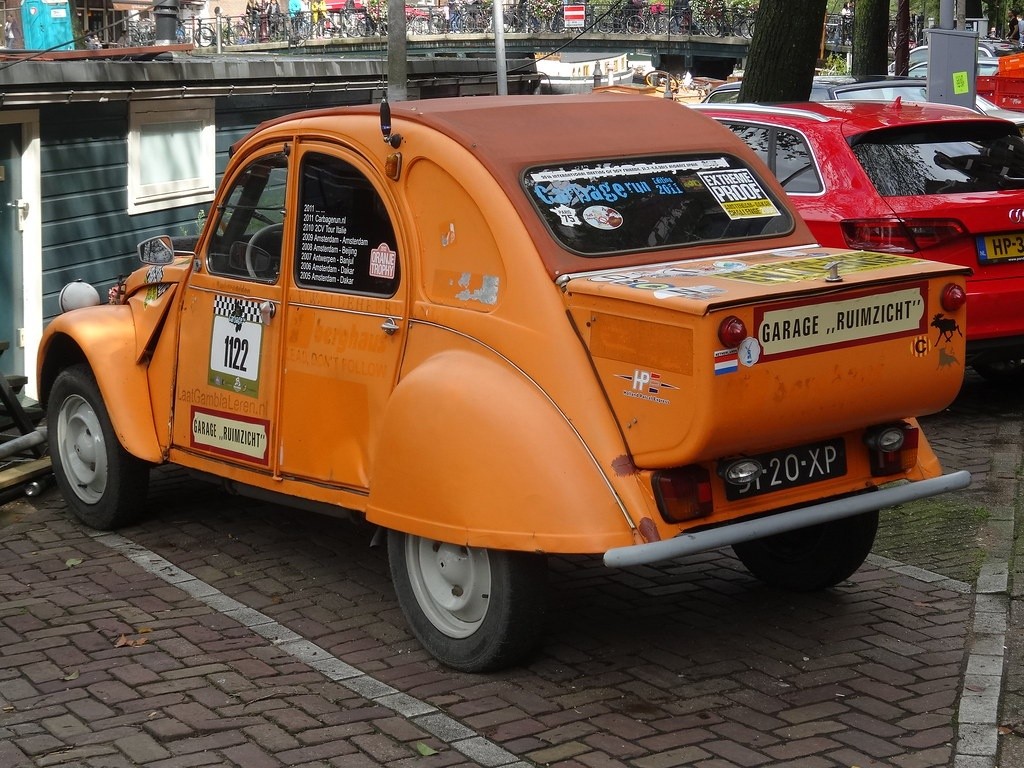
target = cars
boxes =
[700,76,1024,137]
[38,91,973,672]
[682,96,1024,388]
[908,57,999,76]
[888,43,997,76]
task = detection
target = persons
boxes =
[84,30,130,50]
[622,1,692,34]
[4,13,23,49]
[1006,9,1023,45]
[839,0,854,45]
[245,0,359,41]
[449,0,592,34]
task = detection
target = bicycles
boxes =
[645,70,700,93]
[175,0,760,47]
[825,14,924,49]
